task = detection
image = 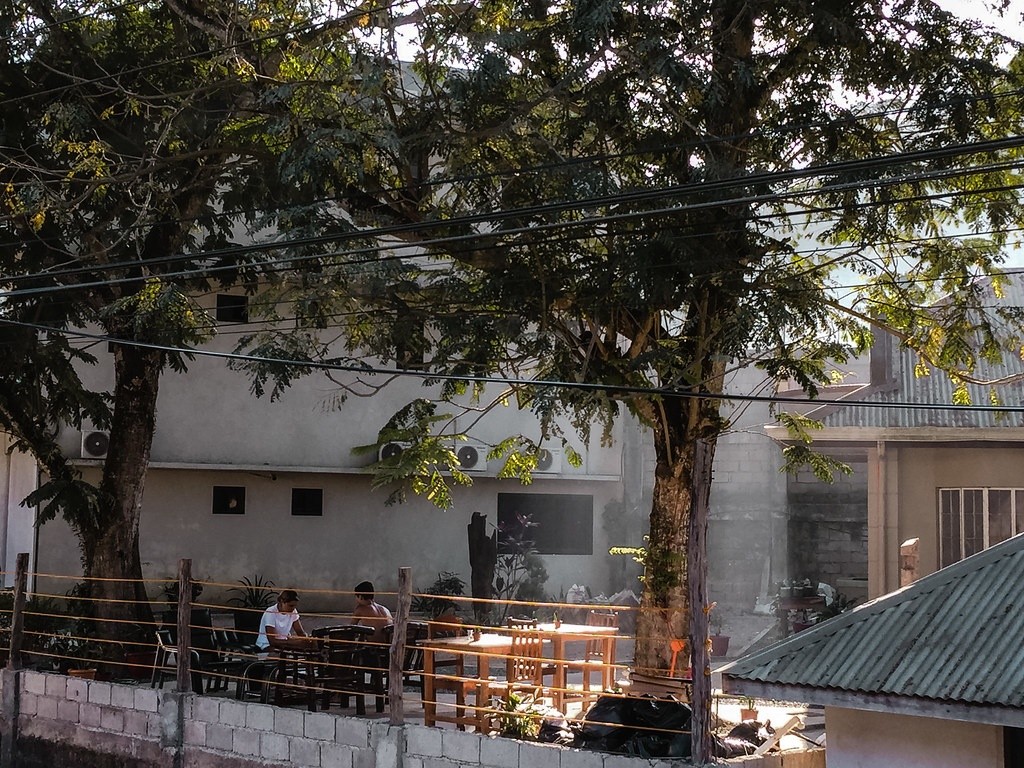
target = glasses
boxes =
[284,601,298,607]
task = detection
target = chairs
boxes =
[290,623,375,715]
[562,609,619,713]
[482,628,545,739]
[226,605,304,688]
[154,603,229,694]
[507,618,568,707]
[372,622,431,712]
[423,614,478,731]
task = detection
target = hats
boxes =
[277,589,297,603]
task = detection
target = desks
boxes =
[271,642,418,711]
[419,622,618,732]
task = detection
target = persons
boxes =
[330,581,395,692]
[253,588,316,693]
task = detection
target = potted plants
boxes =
[225,572,284,641]
[742,696,759,721]
[155,574,213,641]
[709,614,731,658]
[67,656,99,682]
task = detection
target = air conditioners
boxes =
[528,448,564,474]
[378,440,415,467]
[452,444,488,471]
[79,428,111,458]
